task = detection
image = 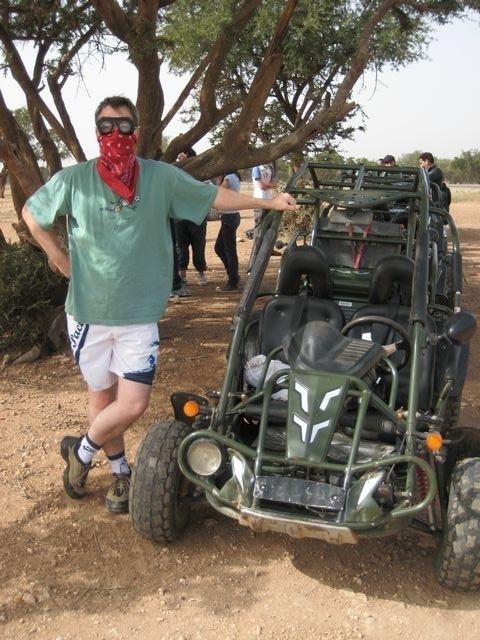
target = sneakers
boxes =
[61,436,92,499]
[105,465,132,514]
[176,273,240,297]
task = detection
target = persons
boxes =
[247,162,278,241]
[375,159,384,178]
[419,152,450,253]
[152,142,245,298]
[380,154,402,206]
[21,92,305,516]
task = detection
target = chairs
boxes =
[255,208,437,408]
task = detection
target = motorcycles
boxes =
[129,160,479,591]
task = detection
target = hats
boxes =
[382,155,395,163]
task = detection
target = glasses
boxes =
[96,116,135,135]
